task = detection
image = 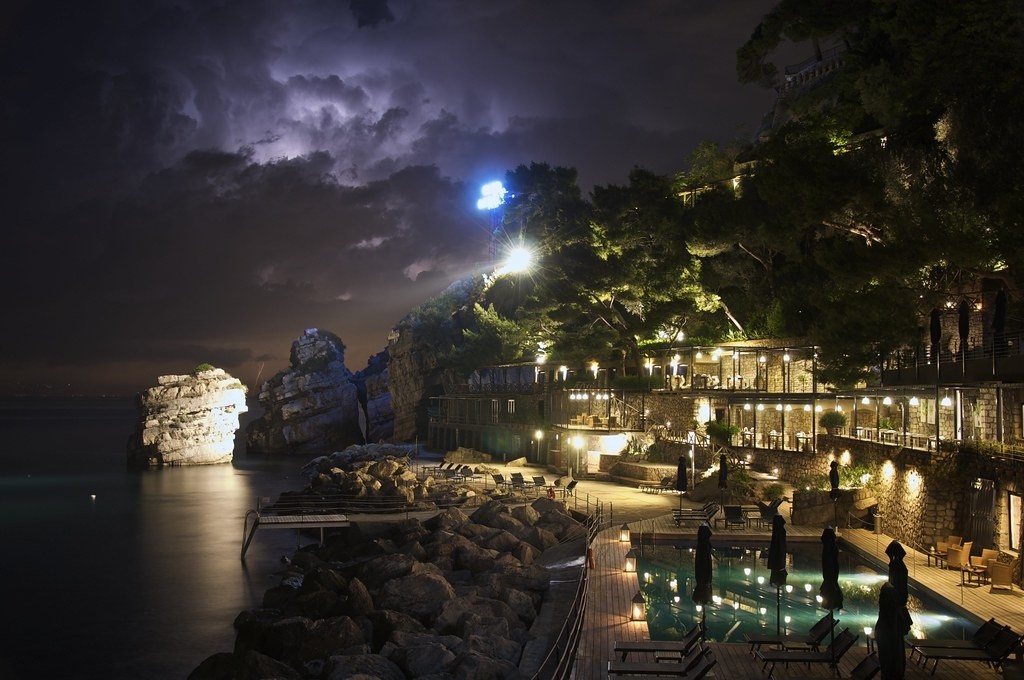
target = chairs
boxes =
[667,373,722,389]
[926,534,1019,592]
[856,427,945,450]
[421,461,579,497]
[606,616,1024,680]
[639,475,783,531]
[569,413,623,428]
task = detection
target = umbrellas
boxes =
[991,289,1005,355]
[767,515,789,636]
[873,582,913,680]
[676,456,687,516]
[829,461,842,518]
[928,309,942,365]
[884,540,908,601]
[718,454,728,513]
[819,528,844,642]
[957,301,971,360]
[691,525,713,644]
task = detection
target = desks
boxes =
[928,552,947,569]
[963,569,986,587]
[742,432,815,453]
